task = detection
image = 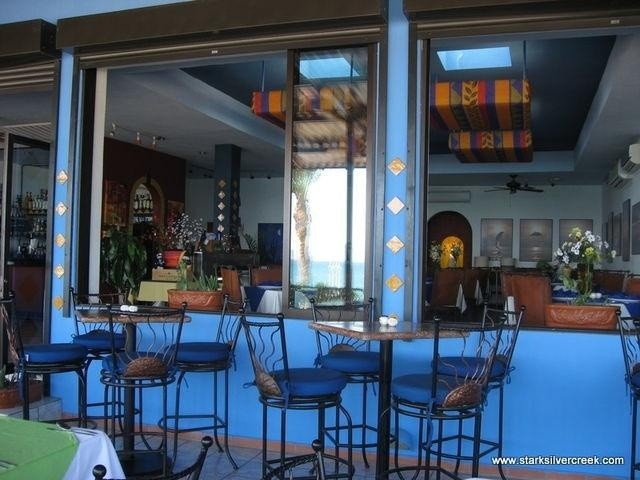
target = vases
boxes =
[546,304,621,331]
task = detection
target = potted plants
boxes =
[167,260,224,311]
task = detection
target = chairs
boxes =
[311,298,404,480]
[615,309,640,480]
[238,306,356,480]
[99,302,188,476]
[430,300,526,479]
[69,287,130,433]
[164,294,246,471]
[4,290,88,425]
[390,316,507,480]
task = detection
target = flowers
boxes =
[553,227,621,305]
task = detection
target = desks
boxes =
[74,300,188,480]
[307,314,471,480]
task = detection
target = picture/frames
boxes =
[613,213,621,256]
[519,218,553,262]
[558,219,593,248]
[622,199,632,262]
[602,223,608,244]
[608,211,613,249]
[632,201,640,255]
[480,218,513,258]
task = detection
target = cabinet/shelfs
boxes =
[10,188,48,321]
[434,265,521,304]
[128,177,164,240]
[207,248,256,286]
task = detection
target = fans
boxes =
[484,174,543,193]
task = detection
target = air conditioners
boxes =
[620,143,640,174]
[607,160,632,194]
